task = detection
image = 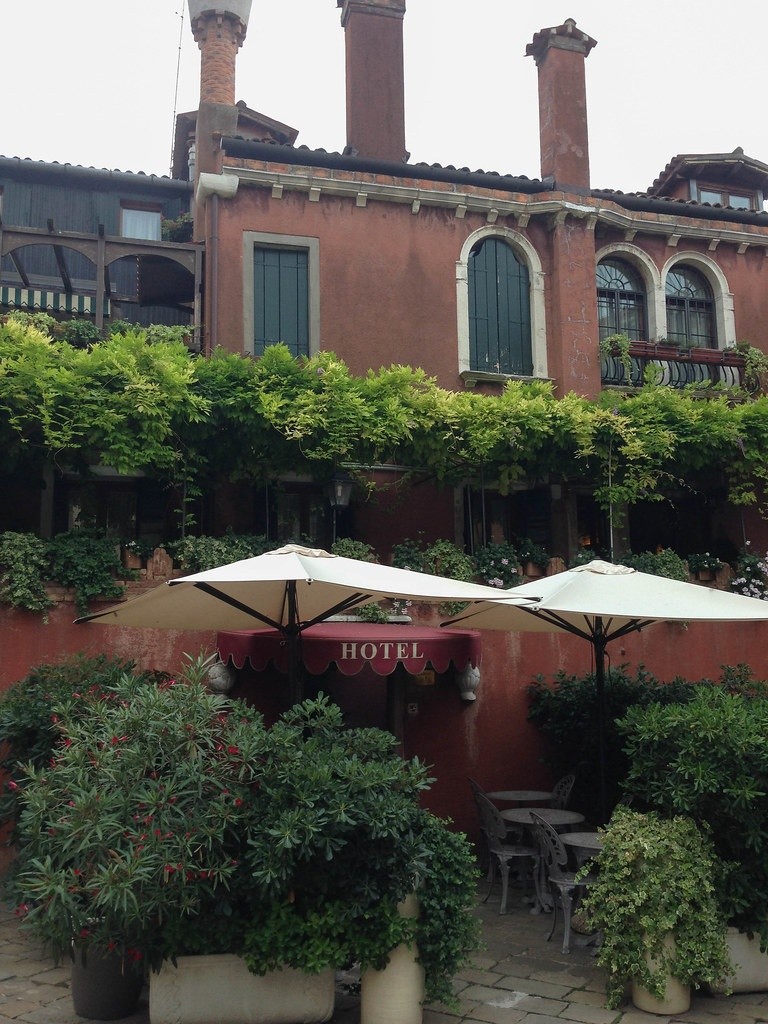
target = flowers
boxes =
[687,552,724,572]
[123,537,154,561]
[8,647,275,978]
[160,207,193,232]
[517,537,551,569]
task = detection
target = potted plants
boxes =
[599,332,768,389]
[576,803,742,1015]
[613,663,768,996]
[149,689,487,1024]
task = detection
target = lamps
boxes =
[322,462,356,516]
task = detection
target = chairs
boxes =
[474,791,542,915]
[528,810,605,957]
[550,775,577,833]
[466,775,523,875]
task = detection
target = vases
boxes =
[69,940,143,1021]
[168,223,192,244]
[696,568,717,581]
[523,562,544,577]
[123,547,142,569]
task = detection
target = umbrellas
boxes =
[439,560,768,819]
[72,544,544,734]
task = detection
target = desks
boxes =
[498,808,586,915]
[558,832,605,946]
[482,791,556,888]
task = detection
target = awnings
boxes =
[215,622,482,676]
